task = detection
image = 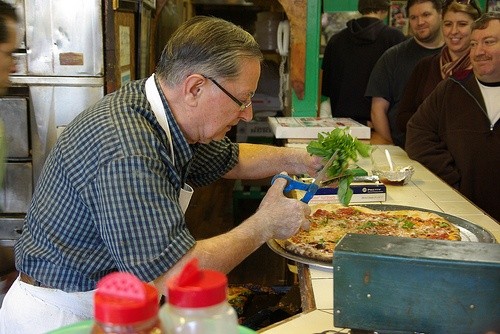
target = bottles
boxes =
[90,272,161,334]
[158,258,238,334]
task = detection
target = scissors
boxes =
[271,148,354,203]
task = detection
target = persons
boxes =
[367,1,446,146]
[0,17,349,334]
[396,0,482,150]
[405,11,500,225]
[322,0,407,131]
[0,1,20,188]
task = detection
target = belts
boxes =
[18,270,51,289]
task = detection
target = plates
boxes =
[266,203,496,270]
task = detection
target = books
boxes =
[269,117,386,206]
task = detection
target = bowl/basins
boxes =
[371,165,415,186]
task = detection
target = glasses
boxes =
[187,72,254,112]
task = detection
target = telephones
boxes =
[277,20,289,57]
